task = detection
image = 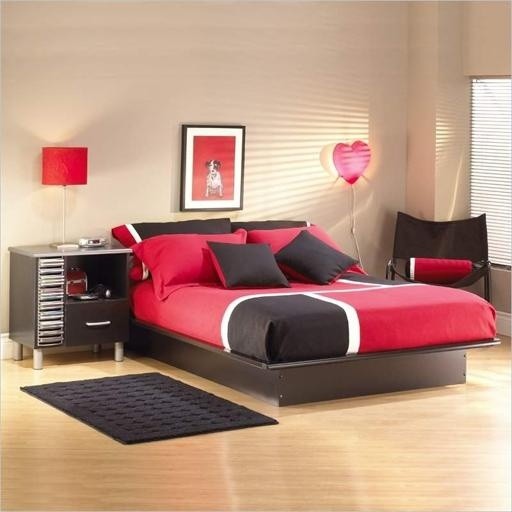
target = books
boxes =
[39,258,65,343]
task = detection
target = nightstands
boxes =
[7,243,133,371]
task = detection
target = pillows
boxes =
[404,256,474,285]
[111,218,231,282]
[231,221,308,233]
[131,227,248,302]
[205,240,291,289]
[274,230,359,286]
[247,223,368,283]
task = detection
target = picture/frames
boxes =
[180,125,245,213]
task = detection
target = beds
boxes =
[114,216,502,407]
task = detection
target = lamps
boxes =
[320,139,377,186]
[41,147,88,248]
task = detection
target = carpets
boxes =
[20,371,279,446]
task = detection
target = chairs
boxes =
[385,211,491,303]
